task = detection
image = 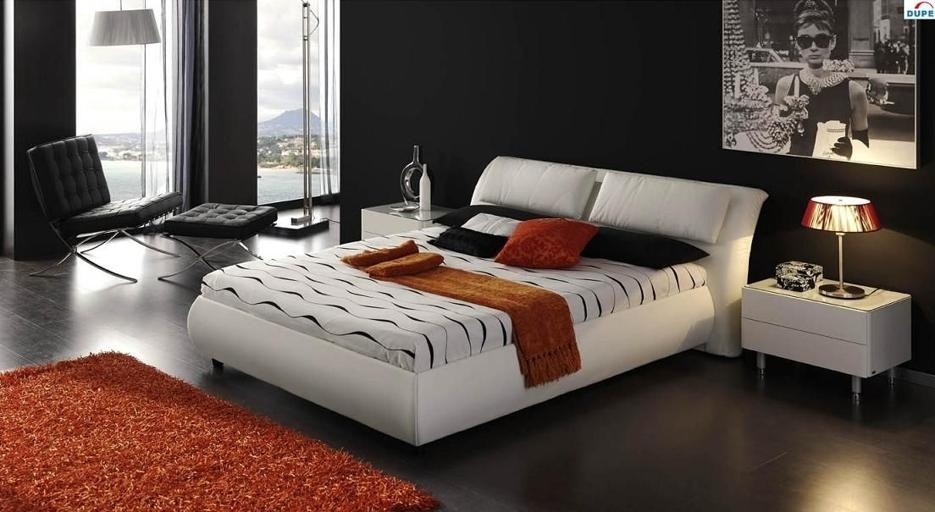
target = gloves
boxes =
[831,129,869,158]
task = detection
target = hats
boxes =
[792,0,838,34]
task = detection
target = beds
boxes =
[184,154,772,451]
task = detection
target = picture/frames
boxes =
[719,0,921,174]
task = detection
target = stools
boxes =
[158,202,278,282]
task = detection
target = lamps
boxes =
[799,193,886,302]
[84,9,173,238]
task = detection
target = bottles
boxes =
[418,163,432,212]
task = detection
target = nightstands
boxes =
[741,272,914,407]
[360,199,462,242]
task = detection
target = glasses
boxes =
[795,34,833,50]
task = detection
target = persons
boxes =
[773,1,870,166]
[873,39,912,74]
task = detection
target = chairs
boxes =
[24,140,183,283]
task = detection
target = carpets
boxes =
[0,351,440,510]
